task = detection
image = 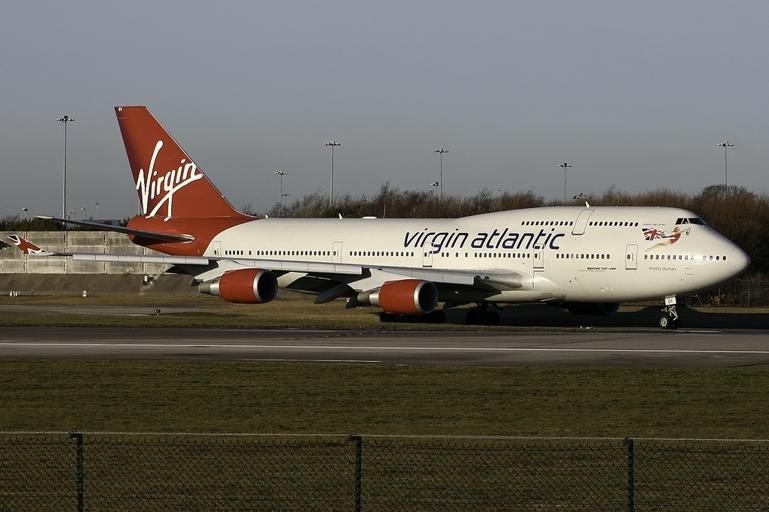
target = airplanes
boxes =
[4,104,754,331]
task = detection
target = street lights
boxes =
[20,114,104,232]
